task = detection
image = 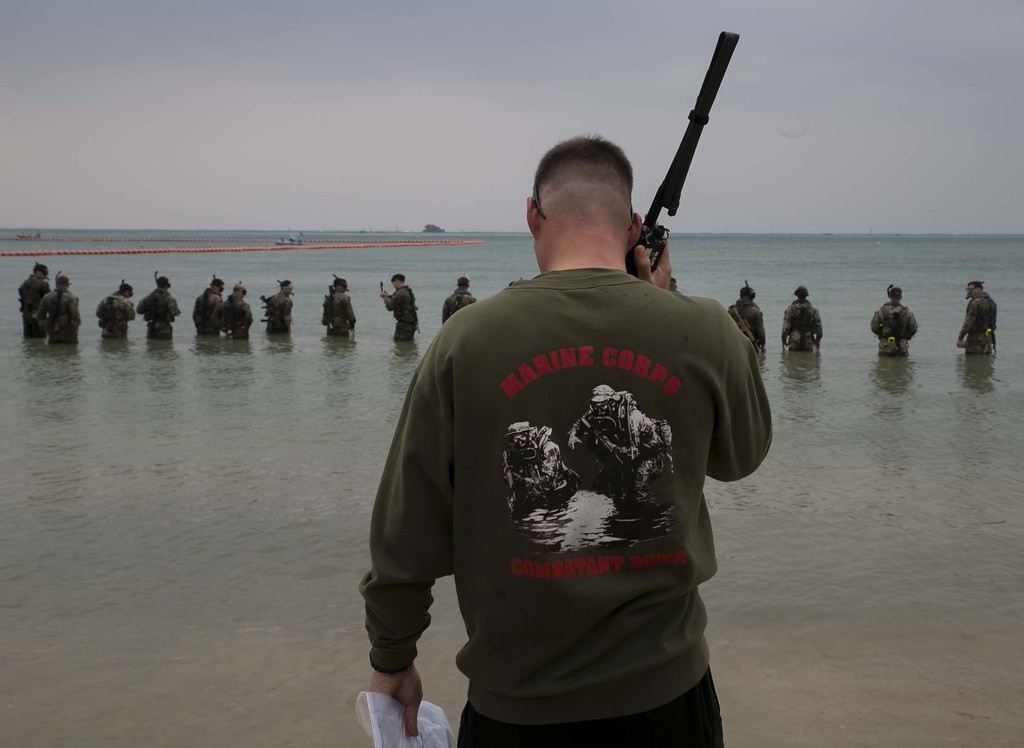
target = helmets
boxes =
[794,286,809,297]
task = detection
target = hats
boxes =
[58,276,69,285]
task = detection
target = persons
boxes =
[670,277,679,291]
[728,280,767,352]
[956,281,997,354]
[358,134,775,748]
[780,286,823,352]
[15,262,513,345]
[870,285,919,356]
